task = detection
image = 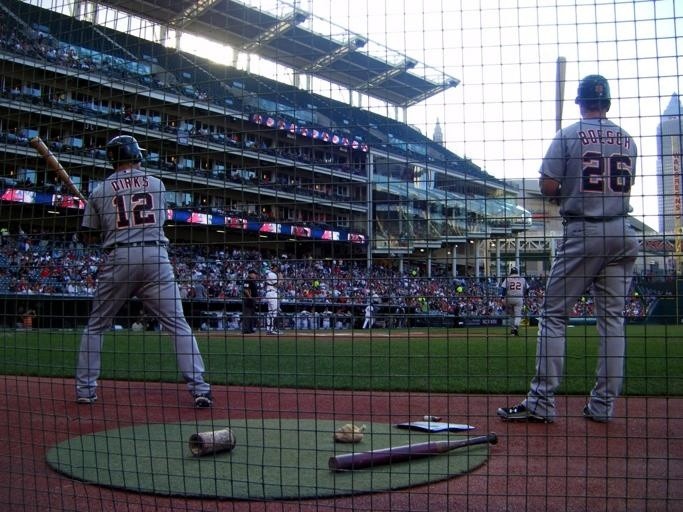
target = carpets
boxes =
[44,418,490,501]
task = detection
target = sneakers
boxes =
[583,403,596,420]
[497,404,552,424]
[511,329,519,336]
[242,329,256,334]
[195,391,213,408]
[266,329,284,336]
[78,394,97,403]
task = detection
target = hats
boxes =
[249,269,258,274]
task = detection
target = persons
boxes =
[73,135,216,407]
[496,73,639,424]
[0,21,682,336]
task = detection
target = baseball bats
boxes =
[556,57,566,135]
[327,432,498,474]
[28,136,89,206]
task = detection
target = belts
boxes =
[104,238,166,254]
[566,212,623,222]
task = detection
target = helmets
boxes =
[511,266,519,272]
[107,135,148,162]
[576,74,611,103]
